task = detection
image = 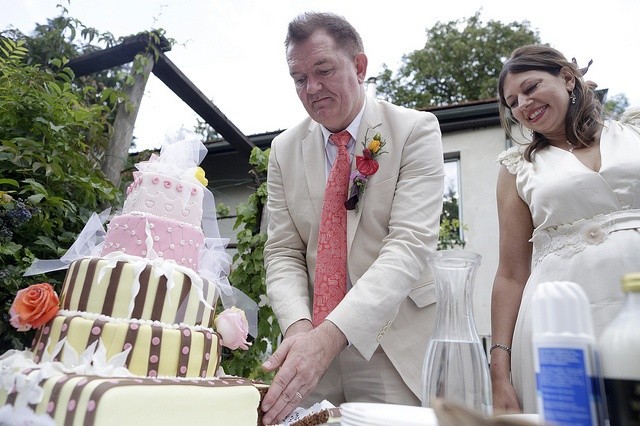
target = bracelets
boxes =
[490,344,511,357]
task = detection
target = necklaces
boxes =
[566,141,575,153]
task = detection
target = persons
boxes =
[491,45,640,426]
[260,12,445,425]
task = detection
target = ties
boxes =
[312,130,352,328]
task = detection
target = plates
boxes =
[336,401,438,424]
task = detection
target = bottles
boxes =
[530,281,607,421]
[421,250,493,419]
[598,274,640,426]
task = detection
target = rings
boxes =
[296,391,302,399]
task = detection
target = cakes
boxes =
[1,124,331,426]
